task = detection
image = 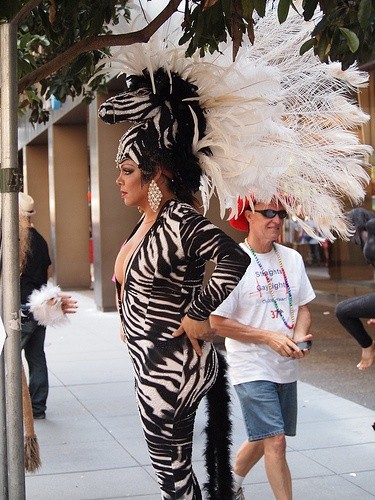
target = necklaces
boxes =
[244,237,296,329]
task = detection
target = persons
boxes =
[335,293,375,370]
[18,191,52,420]
[20,284,77,327]
[211,193,316,500]
[114,121,252,500]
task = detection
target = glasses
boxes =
[250,209,287,219]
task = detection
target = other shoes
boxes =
[32,407,45,419]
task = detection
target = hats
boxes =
[230,189,299,232]
[18,193,37,216]
